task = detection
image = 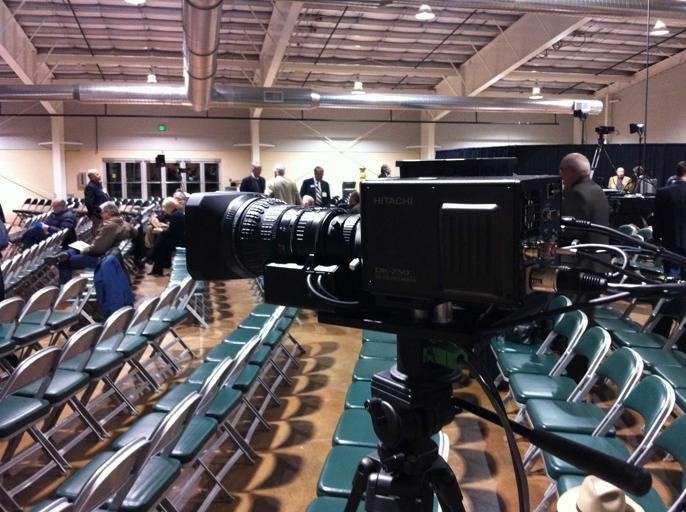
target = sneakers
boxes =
[43,252,68,266]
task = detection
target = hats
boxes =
[556,473,646,512]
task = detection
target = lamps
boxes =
[414,3,435,22]
[529,88,542,100]
[147,74,157,83]
[351,79,365,94]
[648,19,670,37]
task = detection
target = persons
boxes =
[83,169,107,239]
[302,195,314,208]
[300,166,331,209]
[557,152,609,301]
[239,165,266,194]
[652,161,685,354]
[347,191,361,214]
[0,218,7,302]
[140,188,189,275]
[608,165,632,191]
[632,167,651,193]
[378,164,391,178]
[44,201,122,289]
[8,200,76,256]
[272,168,301,206]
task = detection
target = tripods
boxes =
[623,141,663,195]
[589,142,627,195]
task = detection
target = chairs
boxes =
[488,223,684,511]
[304,329,451,511]
[0,193,301,510]
